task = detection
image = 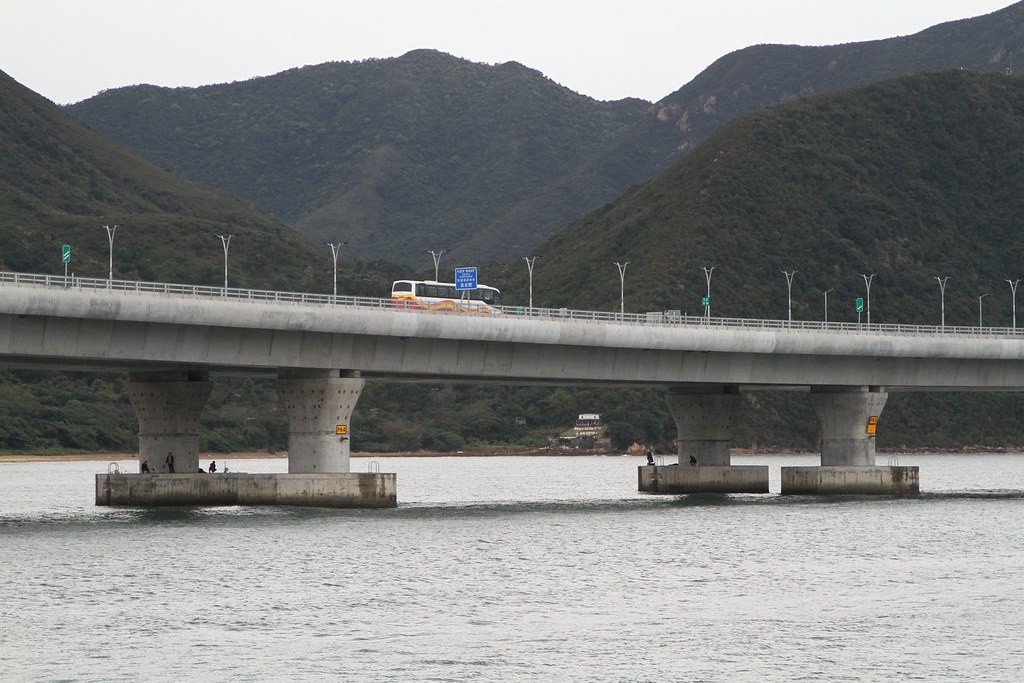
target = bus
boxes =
[391,280,503,314]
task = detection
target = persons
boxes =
[165,451,175,473]
[142,460,150,474]
[646,449,653,466]
[208,460,217,473]
[688,455,697,467]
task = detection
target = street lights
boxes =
[859,274,877,331]
[612,262,634,321]
[825,287,838,330]
[100,224,124,289]
[934,276,954,334]
[980,293,993,335]
[1002,279,1024,335]
[698,266,720,326]
[423,249,452,281]
[520,256,542,316]
[213,233,241,301]
[780,270,800,329]
[323,241,349,305]
